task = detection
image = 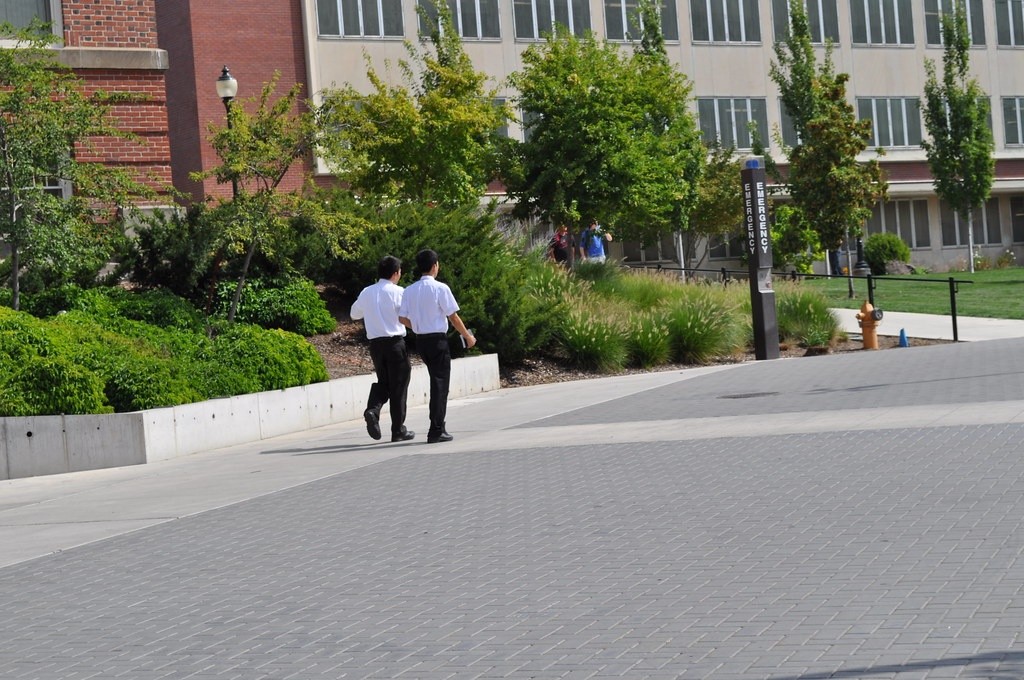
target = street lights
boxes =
[214,67,241,197]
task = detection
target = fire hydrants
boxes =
[856,300,883,350]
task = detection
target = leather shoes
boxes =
[390,430,416,441]
[426,432,453,443]
[363,408,382,440]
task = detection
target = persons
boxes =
[399,250,477,443]
[580,217,613,264]
[549,221,577,270]
[828,239,844,275]
[350,257,415,442]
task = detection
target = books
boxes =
[460,329,475,348]
